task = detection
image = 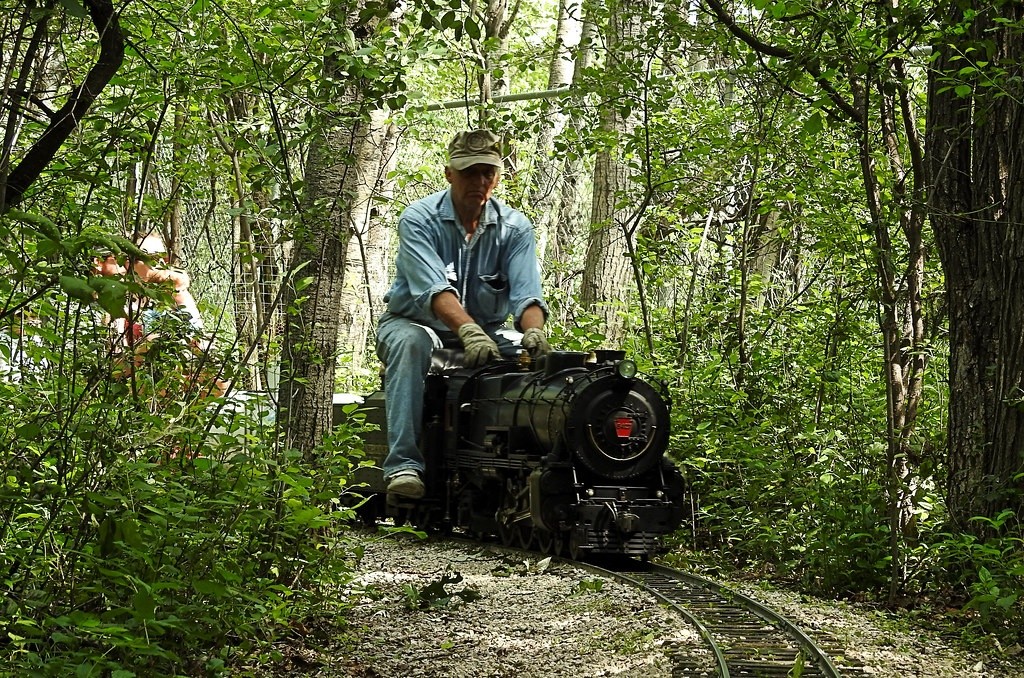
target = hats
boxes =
[448,130,502,170]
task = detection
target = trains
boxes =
[333,339,689,571]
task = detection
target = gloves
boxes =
[522,328,549,357]
[459,324,502,367]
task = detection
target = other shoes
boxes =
[386,469,425,509]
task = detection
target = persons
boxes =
[375,128,551,509]
[90,231,224,459]
